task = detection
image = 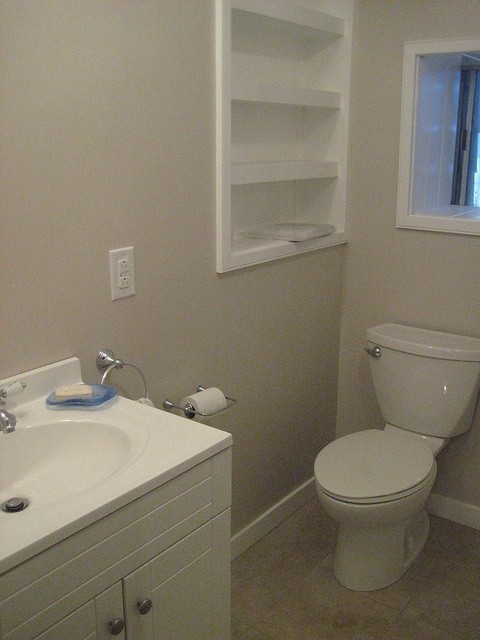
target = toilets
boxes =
[313,323,480,591]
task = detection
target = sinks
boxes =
[0,357,232,571]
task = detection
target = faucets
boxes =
[0,409,17,434]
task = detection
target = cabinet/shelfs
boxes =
[215,0,351,276]
[0,436,230,640]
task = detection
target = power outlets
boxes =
[108,247,137,303]
